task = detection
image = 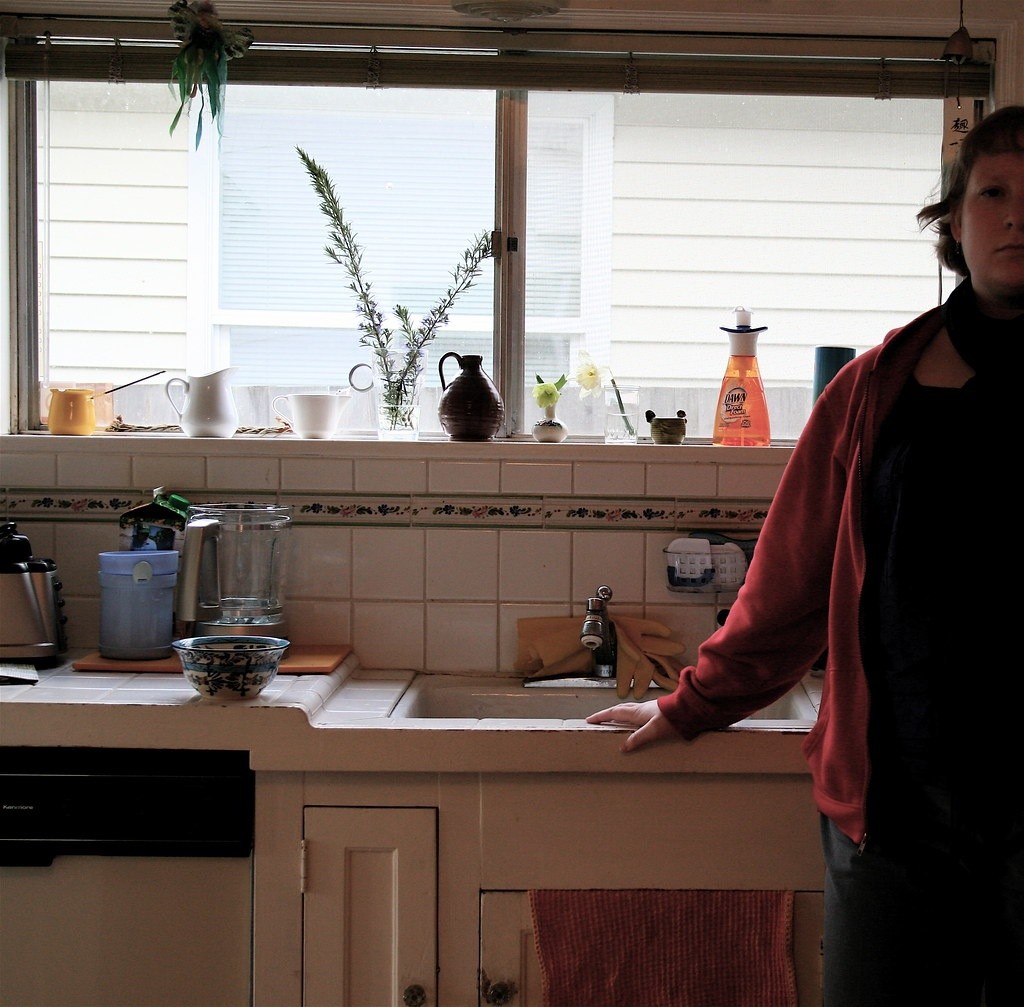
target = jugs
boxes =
[164,366,239,438]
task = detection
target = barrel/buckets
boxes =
[97,571,179,660]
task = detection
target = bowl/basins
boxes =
[172,636,291,702]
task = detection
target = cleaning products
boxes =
[713,307,771,447]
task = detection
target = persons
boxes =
[587,104,1024,1007]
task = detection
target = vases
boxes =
[369,348,430,443]
[531,401,568,441]
[602,383,639,445]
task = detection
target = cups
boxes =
[603,384,640,445]
[272,394,350,438]
[46,388,97,435]
[650,418,686,446]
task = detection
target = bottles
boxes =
[437,352,505,443]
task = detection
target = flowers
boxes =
[532,372,568,410]
[574,348,636,435]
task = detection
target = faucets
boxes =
[578,585,618,678]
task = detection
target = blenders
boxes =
[0,521,69,658]
[179,502,293,659]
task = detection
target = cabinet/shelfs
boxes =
[255,769,823,1007]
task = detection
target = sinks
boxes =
[396,673,817,728]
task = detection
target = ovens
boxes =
[1,744,250,1007]
